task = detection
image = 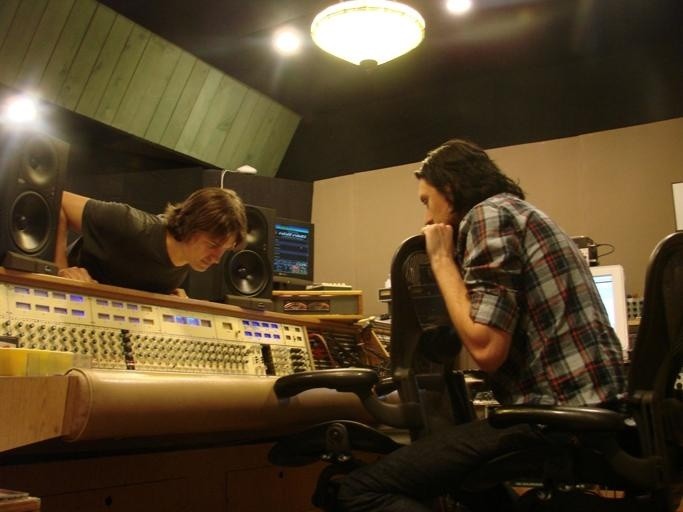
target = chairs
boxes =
[266,231,479,468]
[492,230,683,509]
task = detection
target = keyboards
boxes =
[304,282,352,292]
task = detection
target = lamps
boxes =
[310,1,427,76]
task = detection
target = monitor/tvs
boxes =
[272,216,315,291]
[588,264,630,362]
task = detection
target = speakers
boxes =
[0,124,71,267]
[188,201,276,304]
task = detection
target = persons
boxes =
[51,188,248,299]
[330,139,628,512]
